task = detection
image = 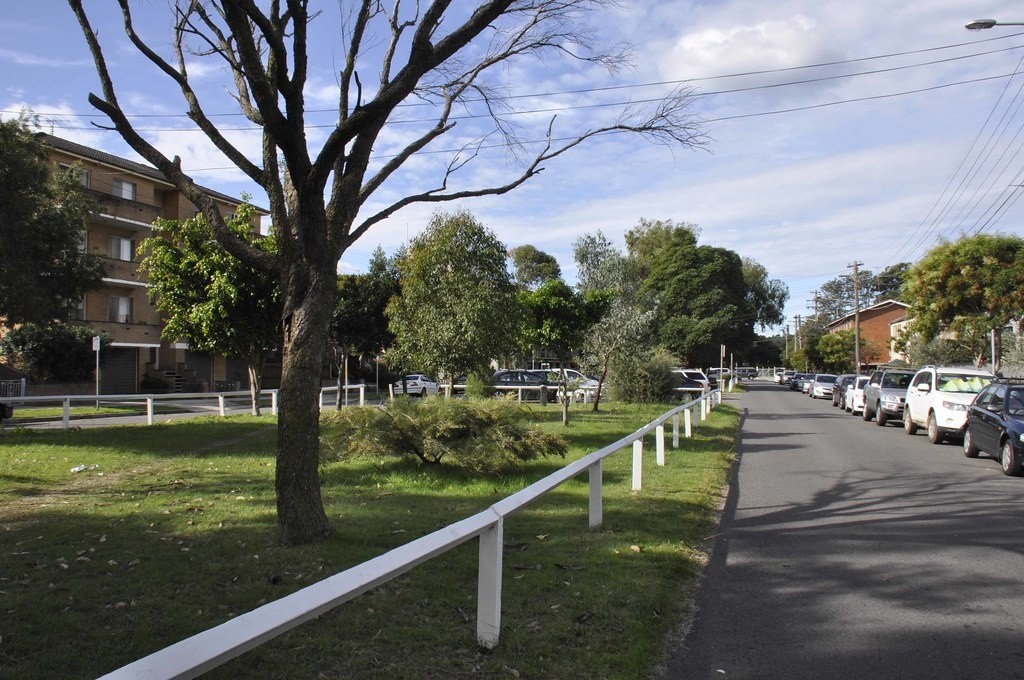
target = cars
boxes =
[963,376,1024,476]
[394,373,443,399]
[452,373,470,394]
[809,373,839,400]
[832,374,860,410]
[664,367,731,406]
[801,374,816,395]
[774,367,799,385]
[526,368,603,402]
[844,374,875,416]
[790,372,807,392]
[480,370,567,403]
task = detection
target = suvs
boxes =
[862,365,922,426]
[733,367,758,381]
[903,364,1004,444]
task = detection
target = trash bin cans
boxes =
[216,381,241,398]
